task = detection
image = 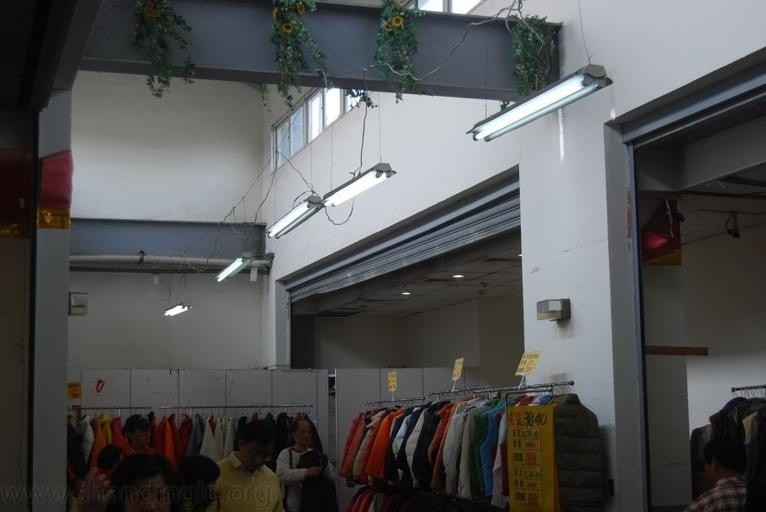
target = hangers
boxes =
[368,396,425,406]
[86,404,286,417]
[430,382,571,395]
[732,385,766,398]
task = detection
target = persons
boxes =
[67,414,221,512]
[686,437,749,512]
[206,423,286,511]
[276,417,337,511]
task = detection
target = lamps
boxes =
[162,270,192,316]
[322,92,396,207]
[465,0,613,142]
[264,87,323,239]
[215,199,249,282]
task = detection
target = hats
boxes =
[126,414,149,431]
